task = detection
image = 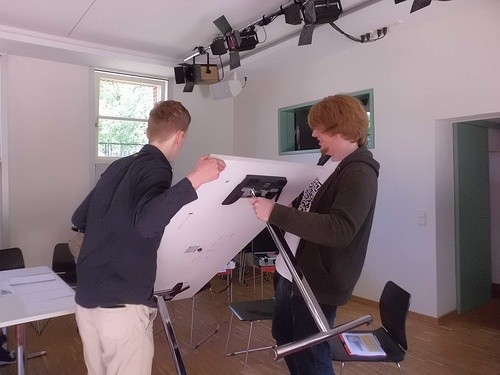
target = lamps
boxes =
[173,0,387,93]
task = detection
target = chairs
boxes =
[238,224,283,287]
[331,281,411,375]
[225,272,277,375]
[0,243,79,336]
[154,282,220,349]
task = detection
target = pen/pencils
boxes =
[374,335,380,345]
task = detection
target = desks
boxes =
[0,266,75,375]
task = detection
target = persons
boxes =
[70,100,227,375]
[250,94,381,375]
